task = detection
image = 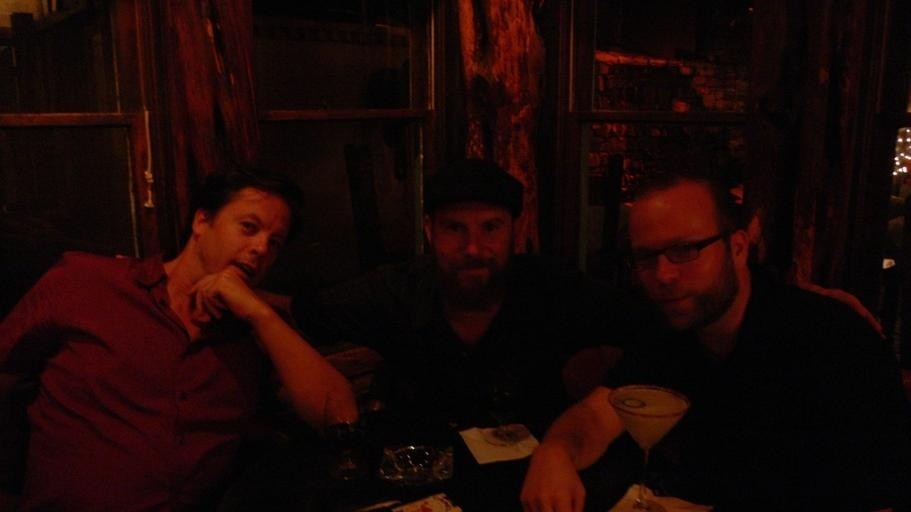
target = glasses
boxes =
[630,230,731,269]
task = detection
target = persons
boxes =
[0,169,359,512]
[254,161,571,451]
[520,153,911,512]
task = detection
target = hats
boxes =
[423,160,525,220]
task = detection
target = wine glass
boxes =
[607,383,691,510]
[326,380,376,479]
